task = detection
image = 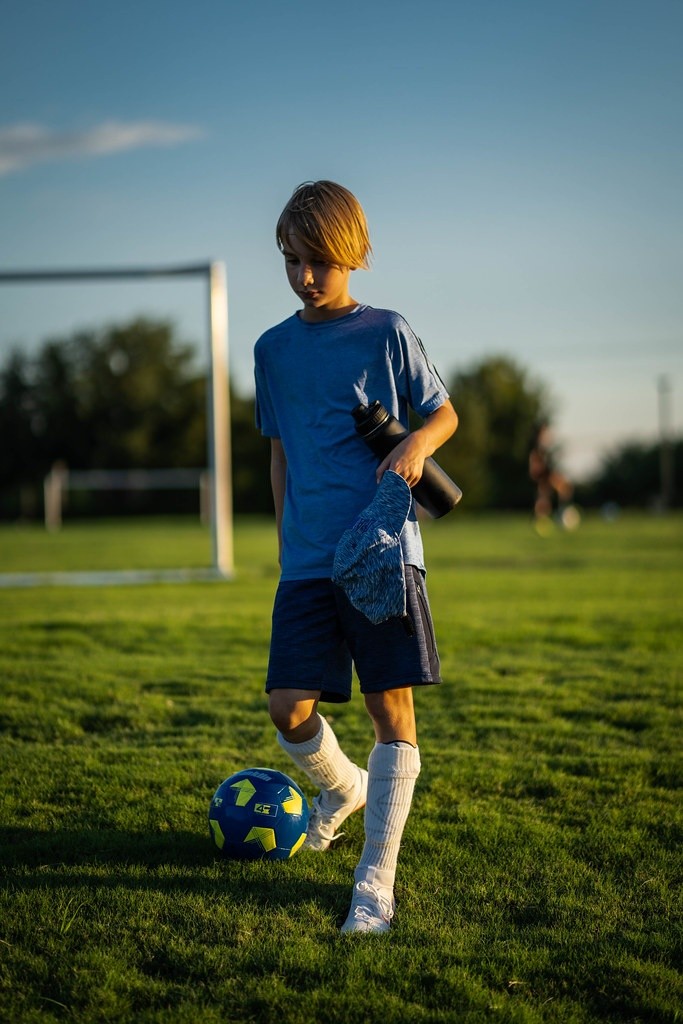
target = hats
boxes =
[331,469,412,625]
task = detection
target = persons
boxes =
[254,180,458,933]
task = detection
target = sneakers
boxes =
[304,767,368,851]
[341,880,394,933]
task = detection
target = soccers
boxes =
[206,767,311,862]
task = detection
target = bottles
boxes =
[350,398,463,519]
